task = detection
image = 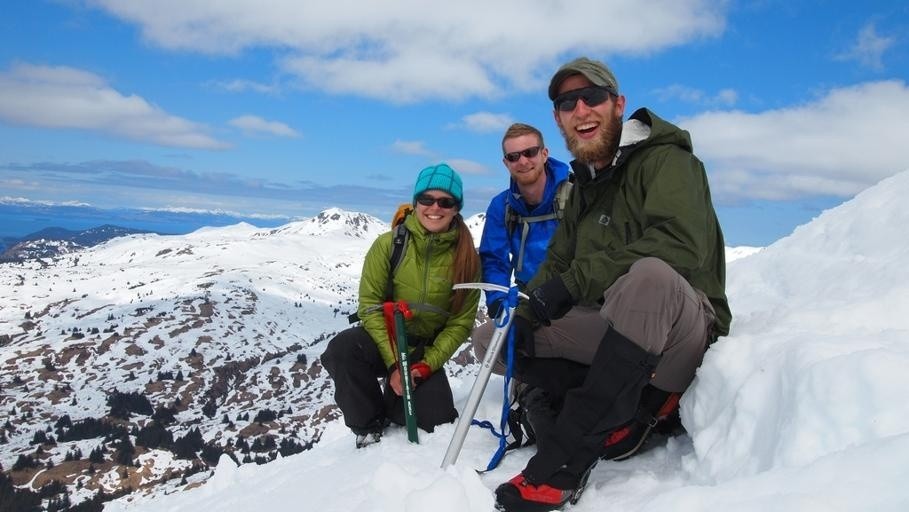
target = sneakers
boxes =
[355,416,391,449]
[600,386,678,463]
[494,452,599,512]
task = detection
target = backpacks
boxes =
[385,203,414,276]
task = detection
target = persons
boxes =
[478,122,576,320]
[471,56,732,511]
[321,163,480,449]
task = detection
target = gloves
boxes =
[527,274,575,327]
[500,316,535,366]
[488,300,504,319]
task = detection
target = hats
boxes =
[412,161,464,212]
[548,57,618,101]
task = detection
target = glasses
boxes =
[553,86,609,111]
[504,145,542,162]
[416,193,457,209]
